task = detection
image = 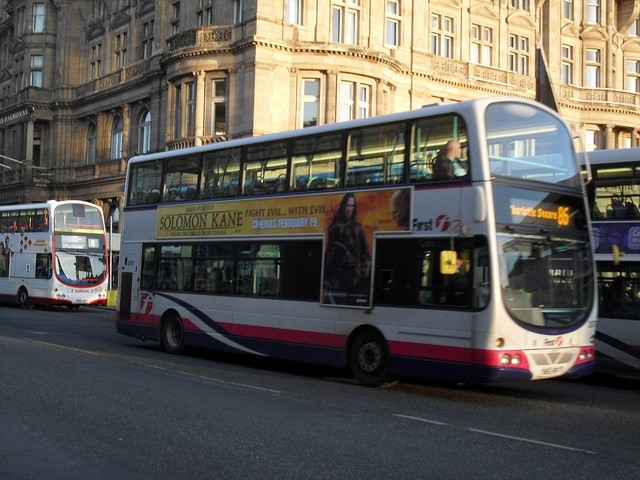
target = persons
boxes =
[323,194,372,305]
[4,236,10,276]
[389,187,409,231]
[606,274,635,305]
[0,241,5,276]
[432,140,469,179]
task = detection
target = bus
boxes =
[447,145,639,388]
[115,95,600,391]
[137,165,433,302]
[0,198,111,311]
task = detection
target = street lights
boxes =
[105,198,114,291]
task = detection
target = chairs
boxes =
[204,173,307,200]
[593,193,640,220]
[129,183,198,205]
